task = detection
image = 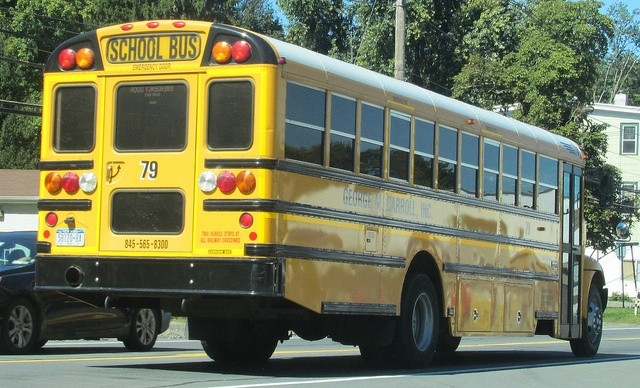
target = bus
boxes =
[33,20,631,369]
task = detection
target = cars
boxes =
[0,231,171,355]
